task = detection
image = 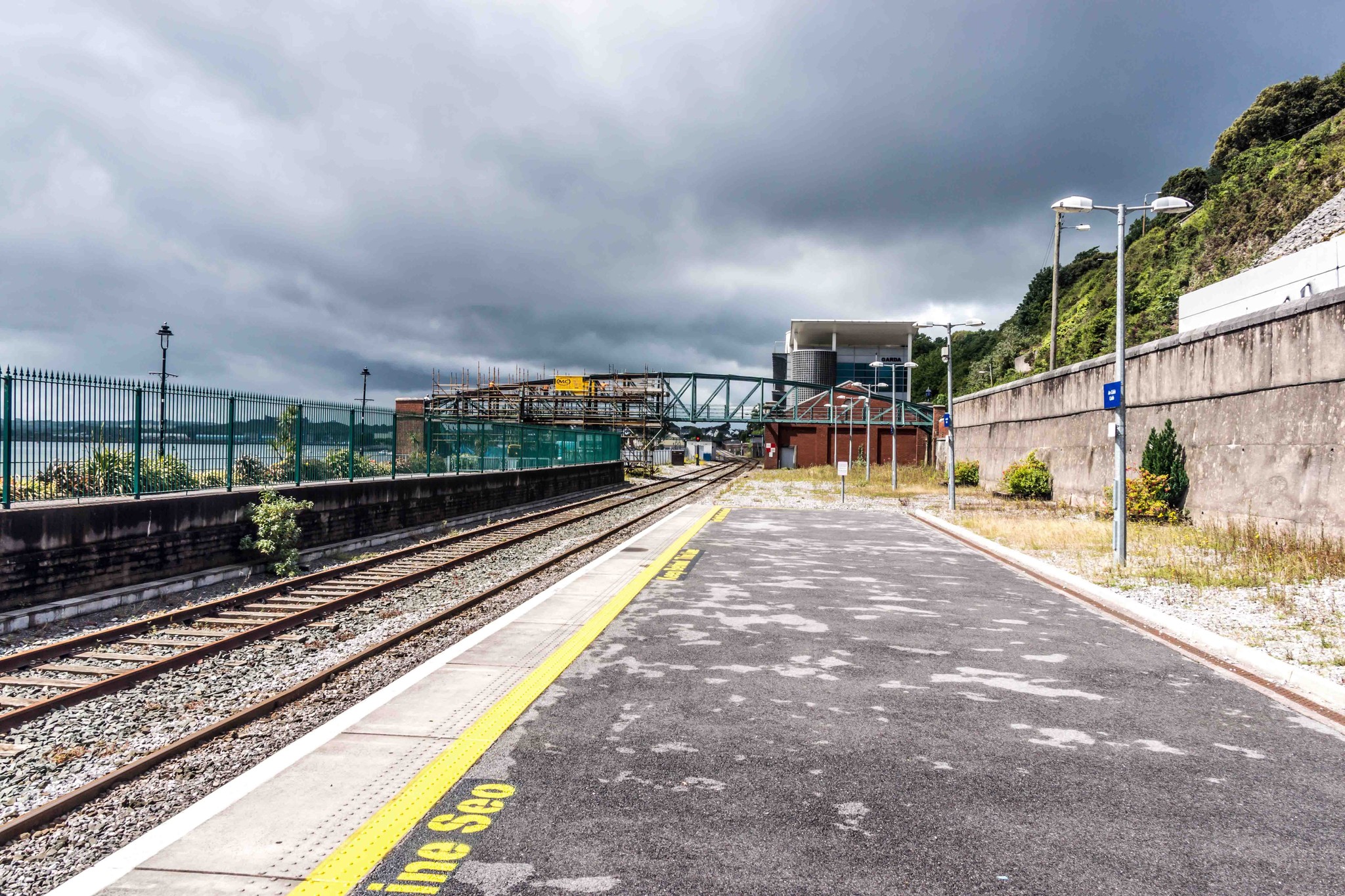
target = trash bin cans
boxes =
[740,450,743,455]
[670,449,685,466]
[703,453,711,462]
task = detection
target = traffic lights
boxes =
[696,437,700,442]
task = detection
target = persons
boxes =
[424,394,431,401]
[598,381,610,396]
[543,384,552,395]
[488,380,495,387]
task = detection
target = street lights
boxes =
[824,404,848,468]
[836,395,866,473]
[869,361,919,490]
[912,318,986,510]
[1050,195,1195,568]
[851,382,889,479]
[148,321,179,457]
[1142,191,1163,237]
[354,366,374,461]
[1049,210,1090,371]
